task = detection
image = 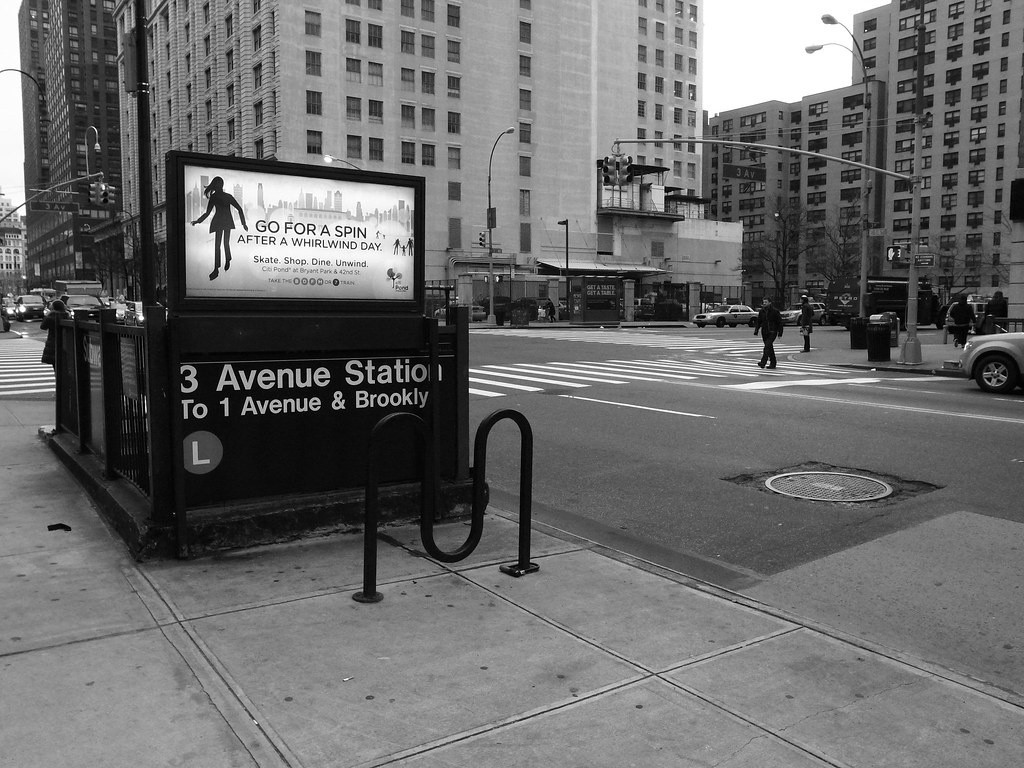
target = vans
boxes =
[823,273,946,332]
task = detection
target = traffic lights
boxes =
[886,245,906,262]
[478,232,486,246]
[601,155,617,187]
[106,186,116,206]
[619,154,633,187]
[98,183,110,205]
[88,181,98,205]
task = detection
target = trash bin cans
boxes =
[495,309,505,326]
[850,317,870,349]
[867,321,891,362]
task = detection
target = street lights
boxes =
[488,127,515,324]
[557,218,570,316]
[806,12,874,317]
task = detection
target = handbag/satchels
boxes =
[800,325,810,336]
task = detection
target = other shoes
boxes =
[766,366,776,369]
[550,321,553,323]
[758,362,765,368]
[954,339,958,347]
[961,344,965,348]
[554,319,556,322]
[800,349,810,353]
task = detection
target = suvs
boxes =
[778,302,828,325]
[944,293,994,336]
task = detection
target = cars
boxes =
[620,298,655,321]
[692,304,760,328]
[124,301,168,326]
[1,279,129,324]
[434,299,487,322]
[961,330,1024,395]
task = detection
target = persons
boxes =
[753,296,783,369]
[40,300,72,372]
[947,291,1008,348]
[543,298,556,323]
[800,297,815,352]
